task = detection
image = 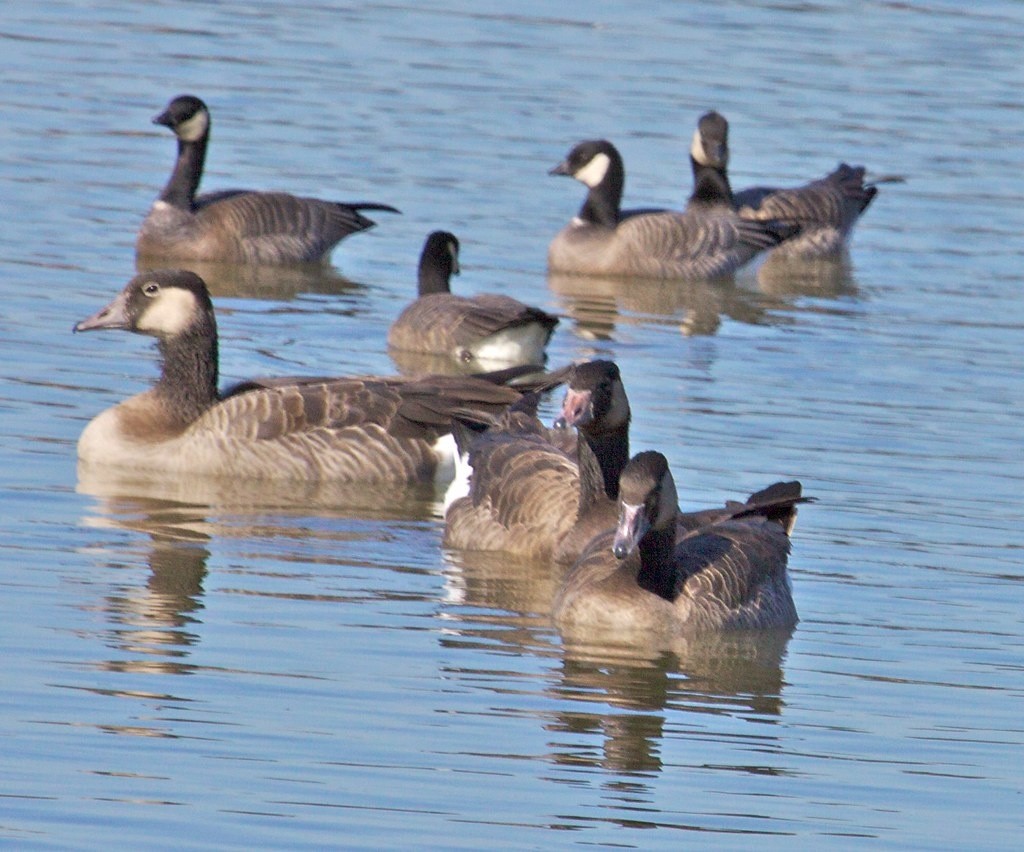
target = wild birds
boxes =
[72,92,880,629]
